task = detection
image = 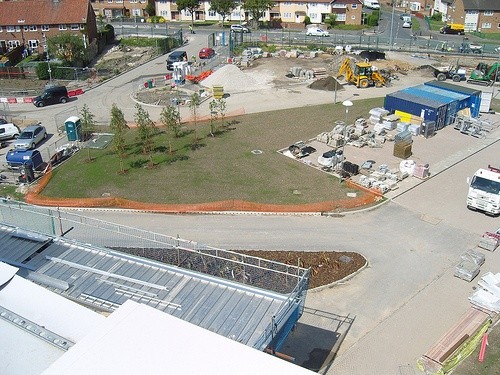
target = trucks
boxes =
[465,169,500,217]
[0,123,20,141]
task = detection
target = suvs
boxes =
[231,24,249,34]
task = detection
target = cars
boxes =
[14,125,48,150]
[399,12,412,28]
[199,47,215,59]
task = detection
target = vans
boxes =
[32,86,68,107]
[366,0,380,10]
[307,28,328,37]
[440,24,464,35]
[165,51,187,71]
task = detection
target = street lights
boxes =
[340,100,353,183]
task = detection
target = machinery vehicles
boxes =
[434,59,499,86]
